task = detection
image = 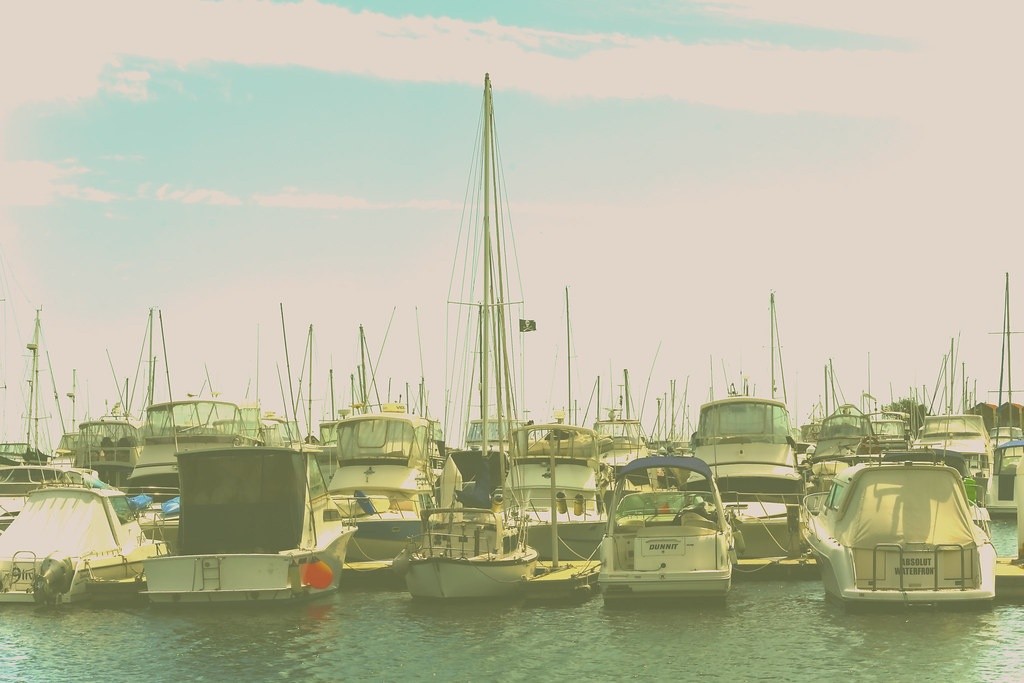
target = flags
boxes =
[519,319,536,332]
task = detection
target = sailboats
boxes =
[0,73,1020,606]
